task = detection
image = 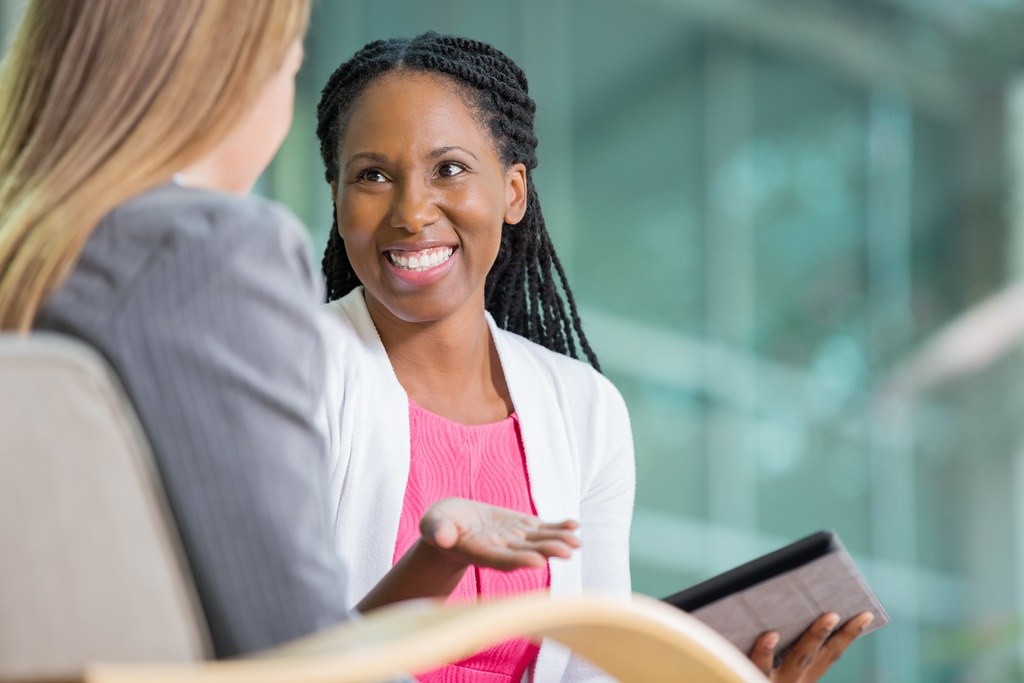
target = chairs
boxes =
[0,330,775,682]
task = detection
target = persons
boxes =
[0,0,359,657]
[310,31,874,682]
